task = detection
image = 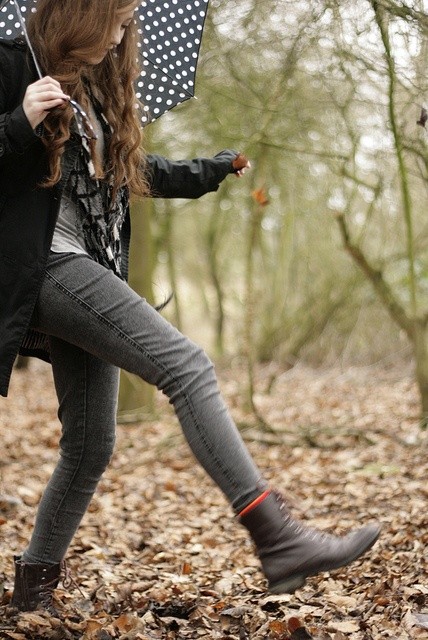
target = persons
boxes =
[2,2,383,622]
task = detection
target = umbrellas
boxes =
[1,2,211,143]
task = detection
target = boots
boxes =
[236,487,381,595]
[9,554,85,623]
